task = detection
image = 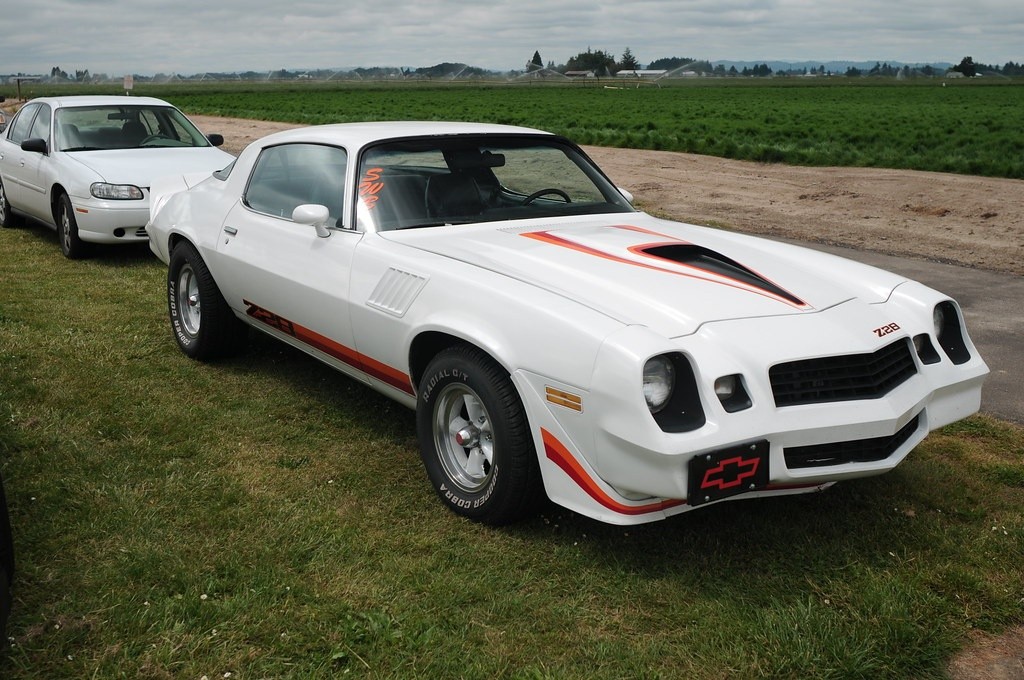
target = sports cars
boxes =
[144,120,991,525]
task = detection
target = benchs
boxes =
[78,127,123,147]
[259,174,427,220]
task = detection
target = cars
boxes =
[0,110,7,135]
[0,95,236,259]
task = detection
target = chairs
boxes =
[62,124,83,147]
[424,173,484,219]
[119,121,149,145]
